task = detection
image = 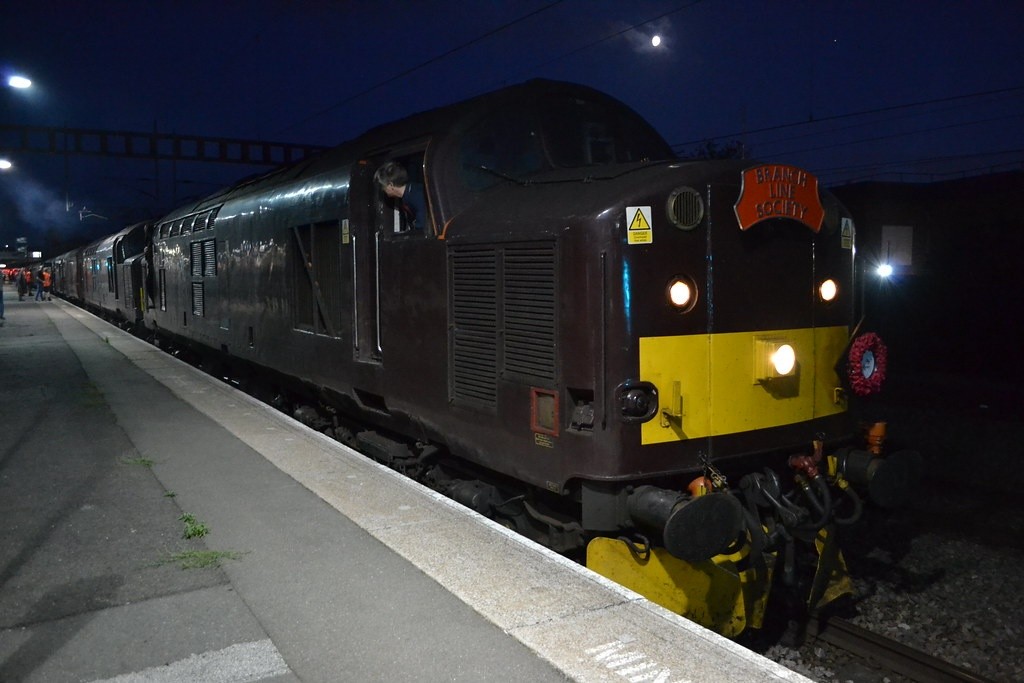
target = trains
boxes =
[25,76,935,638]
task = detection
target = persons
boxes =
[18,269,34,301]
[35,268,52,302]
[373,161,427,229]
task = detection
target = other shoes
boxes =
[0,316,5,319]
[29,293,34,296]
[35,299,38,301]
[48,297,51,301]
[42,297,46,301]
[19,299,25,301]
